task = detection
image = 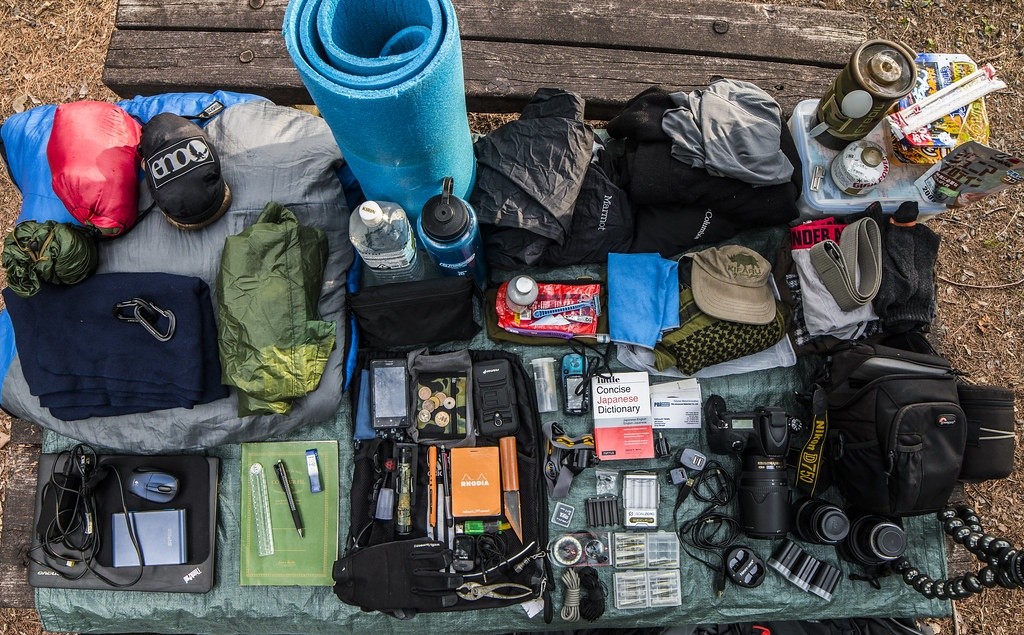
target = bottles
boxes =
[348,201,424,284]
[506,275,538,313]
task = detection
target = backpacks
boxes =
[795,334,1015,517]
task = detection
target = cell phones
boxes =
[370,359,412,428]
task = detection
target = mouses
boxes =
[127,466,179,503]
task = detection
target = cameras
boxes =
[561,353,591,415]
[720,405,810,539]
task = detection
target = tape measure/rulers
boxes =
[248,462,273,557]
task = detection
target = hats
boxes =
[677,245,776,323]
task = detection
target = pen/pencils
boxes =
[440,445,453,528]
[274,460,304,541]
[425,445,437,527]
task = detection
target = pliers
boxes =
[457,582,533,601]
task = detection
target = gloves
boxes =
[332,537,464,621]
[872,224,941,334]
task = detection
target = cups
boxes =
[531,359,558,413]
[417,178,482,279]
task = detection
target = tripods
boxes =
[891,501,1024,601]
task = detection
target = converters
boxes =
[37,472,83,543]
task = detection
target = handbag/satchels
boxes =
[346,348,556,625]
[596,86,804,259]
[0,90,482,454]
